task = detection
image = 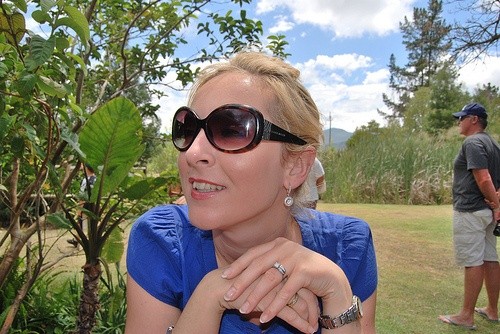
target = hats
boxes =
[452,102,488,119]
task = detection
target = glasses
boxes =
[458,116,469,121]
[172,103,308,154]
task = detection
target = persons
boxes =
[298,158,325,210]
[438,102,500,328]
[122,49,378,334]
[66,160,97,247]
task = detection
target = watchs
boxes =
[318,294,364,329]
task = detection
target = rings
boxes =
[287,292,299,306]
[272,260,288,283]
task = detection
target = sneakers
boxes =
[67,237,78,247]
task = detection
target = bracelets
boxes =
[165,322,175,334]
[492,206,500,211]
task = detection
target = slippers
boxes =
[439,314,476,330]
[475,306,498,321]
